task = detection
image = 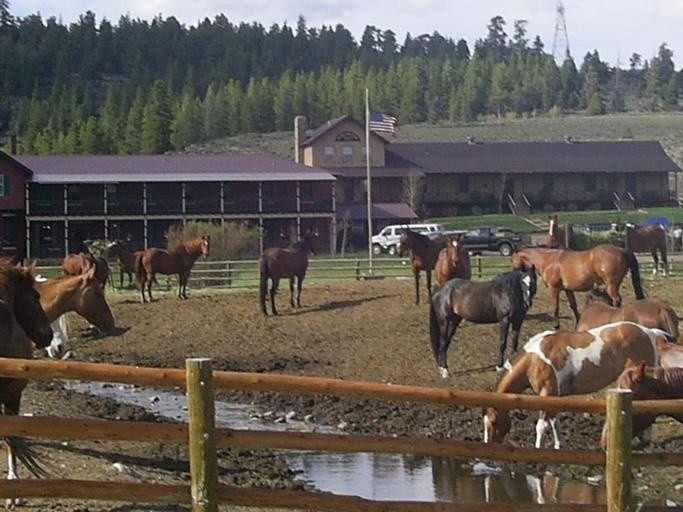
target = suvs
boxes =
[367,220,525,261]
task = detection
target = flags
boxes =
[369,110,397,132]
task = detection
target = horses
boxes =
[482,289,683,450]
[428,263,537,380]
[609,216,667,279]
[258,227,317,316]
[0,258,116,510]
[509,243,645,330]
[61,234,211,302]
[547,213,596,251]
[434,234,471,328]
[397,223,446,305]
